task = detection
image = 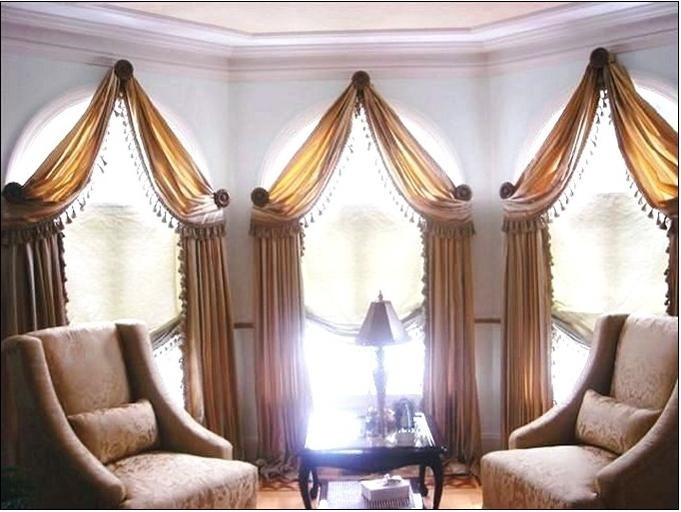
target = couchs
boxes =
[481,312,680,510]
[2,320,258,510]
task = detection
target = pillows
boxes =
[576,391,662,456]
[67,398,164,465]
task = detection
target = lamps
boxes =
[355,290,411,439]
